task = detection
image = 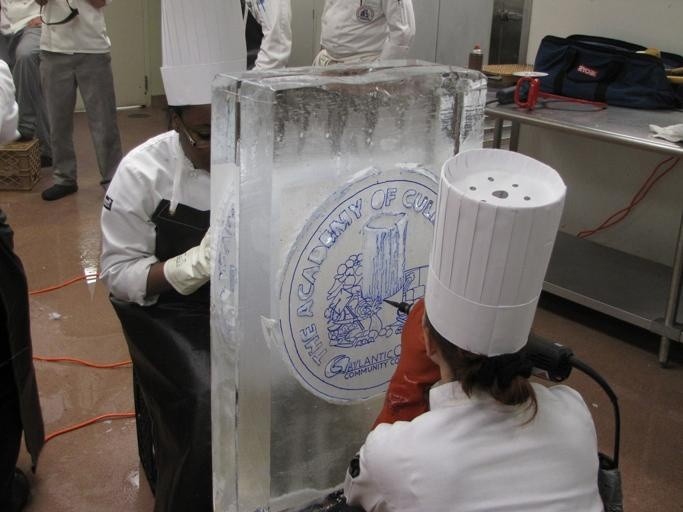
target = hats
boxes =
[156,1,249,108]
[424,148,571,357]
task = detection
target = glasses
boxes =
[173,114,211,150]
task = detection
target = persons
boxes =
[41,1,125,202]
[241,1,294,74]
[0,57,23,156]
[0,1,56,168]
[96,106,214,511]
[343,295,608,511]
[312,0,417,67]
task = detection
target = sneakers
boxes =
[41,155,55,166]
[41,183,77,202]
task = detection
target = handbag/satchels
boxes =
[533,34,682,112]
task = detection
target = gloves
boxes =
[371,297,442,430]
[162,226,211,298]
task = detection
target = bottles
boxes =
[468,43,484,70]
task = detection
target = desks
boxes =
[483,63,683,368]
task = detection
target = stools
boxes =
[0,138,42,191]
